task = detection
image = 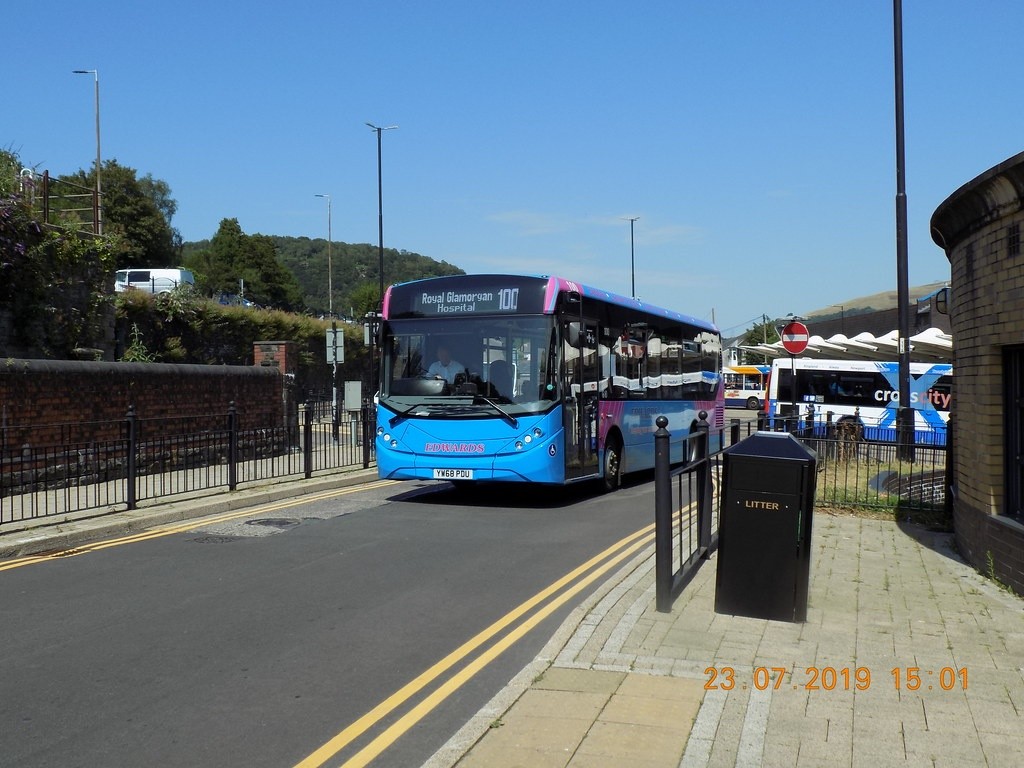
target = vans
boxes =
[111,268,194,300]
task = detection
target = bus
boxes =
[722,362,772,411]
[362,271,727,491]
[769,357,953,449]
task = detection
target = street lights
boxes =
[620,216,641,298]
[71,68,102,250]
[314,193,334,321]
[827,304,844,335]
[363,121,399,310]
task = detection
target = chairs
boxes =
[485,360,512,398]
[568,366,603,401]
[691,389,708,401]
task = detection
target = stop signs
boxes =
[780,322,811,355]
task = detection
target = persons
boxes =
[426,345,467,396]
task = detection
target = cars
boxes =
[216,289,263,310]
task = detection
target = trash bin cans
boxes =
[713,430,819,624]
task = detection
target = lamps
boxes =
[936,288,952,315]
